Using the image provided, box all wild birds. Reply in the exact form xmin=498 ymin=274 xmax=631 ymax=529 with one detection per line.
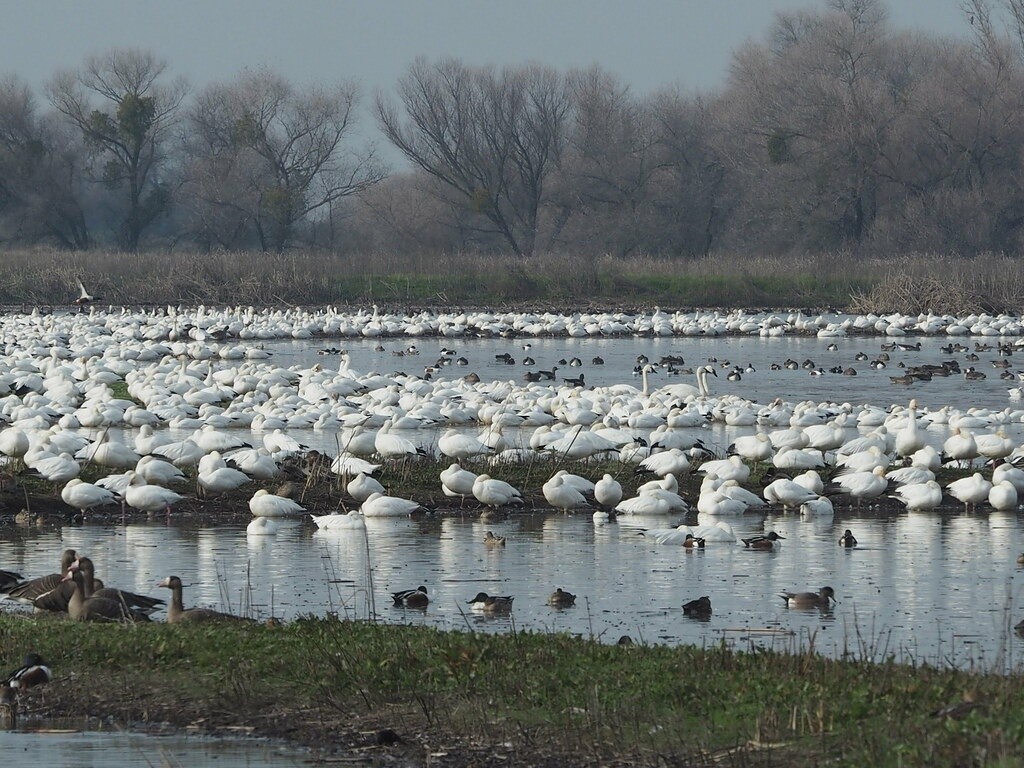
xmin=465 ymin=592 xmax=515 ymax=612
xmin=0 ymin=270 xmax=1024 ymax=551
xmin=548 ymin=588 xmax=577 ymax=604
xmin=390 ymin=586 xmax=429 ymax=610
xmin=777 ymin=587 xmax=836 ymax=609
xmin=0 ymin=549 xmax=256 ymax=629
xmin=618 ymin=636 xmax=634 ymax=649
xmin=0 ymin=653 xmax=52 ymax=700
xmin=681 ymin=597 xmax=712 ymax=621
